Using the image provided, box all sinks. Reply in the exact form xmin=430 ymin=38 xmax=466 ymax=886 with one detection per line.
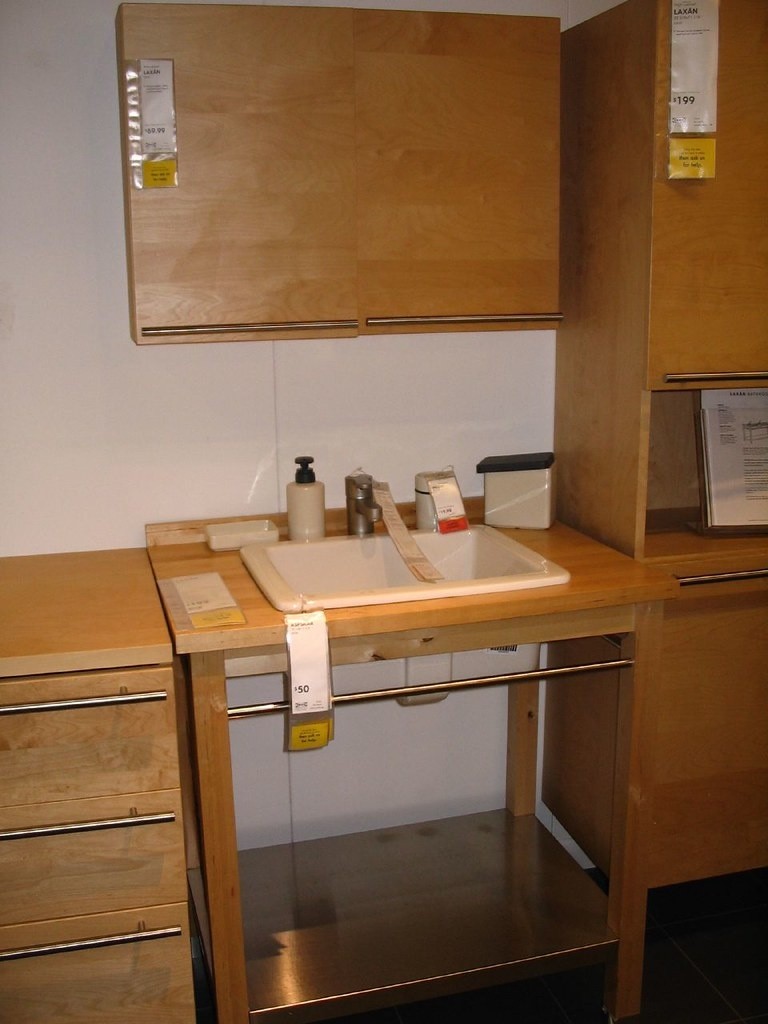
xmin=240 ymin=524 xmax=571 ymax=612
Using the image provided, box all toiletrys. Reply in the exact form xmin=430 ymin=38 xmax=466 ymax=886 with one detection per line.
xmin=286 ymin=456 xmax=325 ymax=540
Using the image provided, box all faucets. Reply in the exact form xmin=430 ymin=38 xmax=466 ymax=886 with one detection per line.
xmin=345 ymin=474 xmax=383 ymax=536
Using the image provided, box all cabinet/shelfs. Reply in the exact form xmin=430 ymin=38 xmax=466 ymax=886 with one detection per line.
xmin=114 ymin=3 xmax=563 ymax=345
xmin=554 ymin=0 xmax=768 ymax=890
xmin=0 ymin=547 xmax=197 ymax=1024
